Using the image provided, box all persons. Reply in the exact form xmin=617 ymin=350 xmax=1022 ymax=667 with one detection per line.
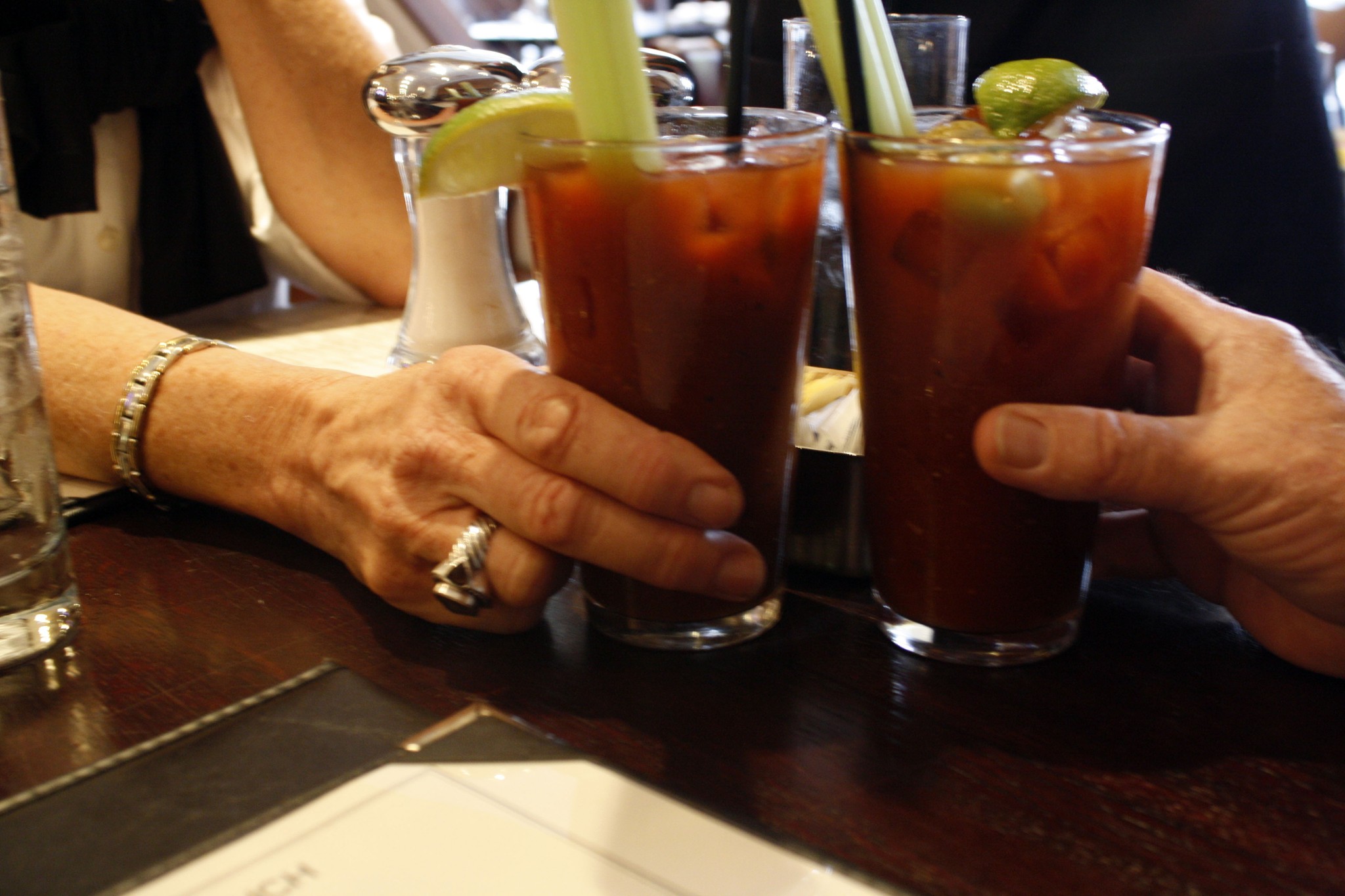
xmin=0 ymin=1 xmax=1345 ymax=683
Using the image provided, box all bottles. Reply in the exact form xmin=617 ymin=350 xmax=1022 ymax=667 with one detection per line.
xmin=365 ymin=47 xmax=545 ymax=377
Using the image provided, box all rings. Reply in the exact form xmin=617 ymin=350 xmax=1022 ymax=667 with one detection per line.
xmin=430 ymin=514 xmax=499 ymax=619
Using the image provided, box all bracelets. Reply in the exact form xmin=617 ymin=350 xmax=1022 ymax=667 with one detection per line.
xmin=108 ymin=336 xmax=235 ymax=502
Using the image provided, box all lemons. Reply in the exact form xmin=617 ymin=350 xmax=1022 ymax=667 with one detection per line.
xmin=417 ymin=86 xmax=591 ymax=198
xmin=974 ymin=57 xmax=1108 ymax=137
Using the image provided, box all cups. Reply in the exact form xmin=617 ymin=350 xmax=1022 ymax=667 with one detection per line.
xmin=780 ymin=12 xmax=969 ymax=370
xmin=518 ymin=104 xmax=832 ymax=649
xmin=833 ymin=109 xmax=1169 ymax=666
xmin=0 ymin=122 xmax=80 ymax=668
